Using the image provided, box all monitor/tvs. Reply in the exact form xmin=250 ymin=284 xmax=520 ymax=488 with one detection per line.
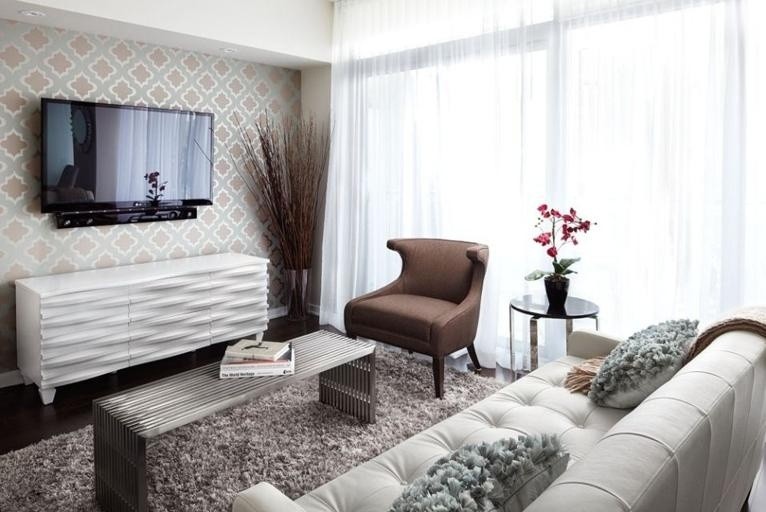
xmin=40 ymin=96 xmax=214 ymax=213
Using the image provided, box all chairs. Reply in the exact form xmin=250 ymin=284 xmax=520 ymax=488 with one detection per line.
xmin=342 ymin=236 xmax=489 ymax=402
xmin=46 ymin=162 xmax=79 ymax=191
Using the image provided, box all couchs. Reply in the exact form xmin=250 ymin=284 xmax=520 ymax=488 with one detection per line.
xmin=226 ymin=308 xmax=763 ymax=512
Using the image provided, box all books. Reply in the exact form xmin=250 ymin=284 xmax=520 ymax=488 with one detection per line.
xmin=218 ymin=339 xmax=295 ymax=380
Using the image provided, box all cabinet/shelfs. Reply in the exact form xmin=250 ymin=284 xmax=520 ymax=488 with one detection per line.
xmin=11 ymin=251 xmax=271 ymax=408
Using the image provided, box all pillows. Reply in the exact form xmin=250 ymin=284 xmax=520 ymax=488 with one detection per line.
xmin=390 ymin=433 xmax=570 ymax=512
xmin=56 ymin=185 xmax=88 ymax=201
xmin=587 ymin=315 xmax=699 ymax=412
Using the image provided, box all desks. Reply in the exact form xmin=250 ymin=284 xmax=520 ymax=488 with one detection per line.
xmin=87 ymin=326 xmax=376 ymax=512
xmin=506 ymin=296 xmax=600 ymax=382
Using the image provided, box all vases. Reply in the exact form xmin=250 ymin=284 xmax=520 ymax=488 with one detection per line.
xmin=542 ymin=275 xmax=569 ymax=309
xmin=284 ymin=266 xmax=313 ymax=322
xmin=149 ymin=200 xmax=161 ymax=206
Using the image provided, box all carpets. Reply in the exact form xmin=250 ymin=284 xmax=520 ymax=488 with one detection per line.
xmin=0 ymin=343 xmax=504 ymax=512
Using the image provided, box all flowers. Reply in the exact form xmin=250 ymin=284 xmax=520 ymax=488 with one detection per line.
xmin=519 ymin=201 xmax=593 ymax=281
xmin=142 ymin=168 xmax=167 ymax=202
